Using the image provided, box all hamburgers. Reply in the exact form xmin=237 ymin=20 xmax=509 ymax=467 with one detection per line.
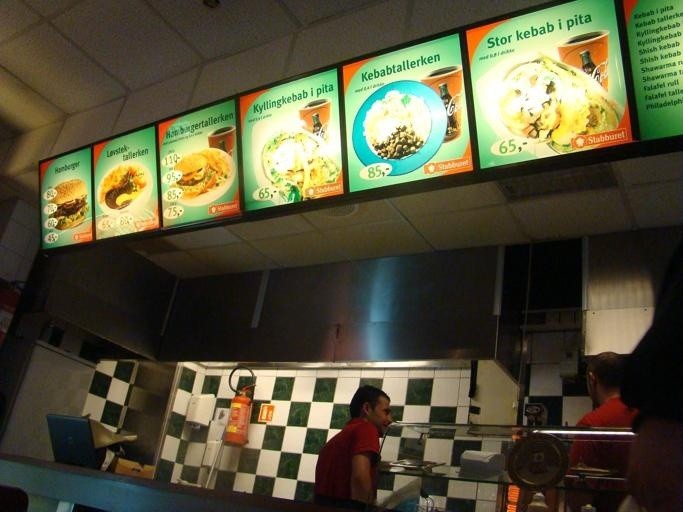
xmin=200 ymin=148 xmax=232 ymax=184
xmin=175 ymin=153 xmax=208 ymax=197
xmin=48 ymin=179 xmax=88 ymax=230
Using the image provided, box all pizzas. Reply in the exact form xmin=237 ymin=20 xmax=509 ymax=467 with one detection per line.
xmin=263 ymin=132 xmax=341 ymax=198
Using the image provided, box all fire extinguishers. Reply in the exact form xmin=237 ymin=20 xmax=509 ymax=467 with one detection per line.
xmin=222 ymin=366 xmax=256 ymax=447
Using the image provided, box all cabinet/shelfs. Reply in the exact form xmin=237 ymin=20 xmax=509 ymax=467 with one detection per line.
xmin=374 ymin=421 xmax=635 ymax=512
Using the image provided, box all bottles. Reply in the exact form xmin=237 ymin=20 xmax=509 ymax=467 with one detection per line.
xmin=437 ymin=84 xmax=461 ymax=141
xmin=579 ymin=50 xmax=601 ymax=82
xmin=311 ymin=113 xmax=323 ymax=138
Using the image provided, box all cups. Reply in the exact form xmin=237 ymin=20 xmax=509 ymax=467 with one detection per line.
xmin=298 ymin=98 xmax=332 ymax=142
xmin=423 ymin=64 xmax=461 ymax=142
xmin=207 ymin=126 xmax=236 ymax=158
xmin=558 ymin=28 xmax=610 ymax=93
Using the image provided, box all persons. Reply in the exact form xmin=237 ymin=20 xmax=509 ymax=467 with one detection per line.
xmin=311 ymin=383 xmax=391 ymax=505
xmin=566 ymin=350 xmax=642 ymax=510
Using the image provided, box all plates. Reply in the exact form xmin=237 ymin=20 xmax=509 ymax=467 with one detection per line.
xmin=170 ymin=147 xmax=235 ymax=208
xmin=97 ymin=161 xmax=154 ymax=214
xmin=487 ymin=54 xmax=625 ymax=154
xmin=352 ymin=80 xmax=448 ymax=175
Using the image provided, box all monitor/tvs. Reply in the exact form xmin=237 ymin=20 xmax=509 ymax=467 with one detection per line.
xmin=46 ymin=414 xmax=107 ymax=470
